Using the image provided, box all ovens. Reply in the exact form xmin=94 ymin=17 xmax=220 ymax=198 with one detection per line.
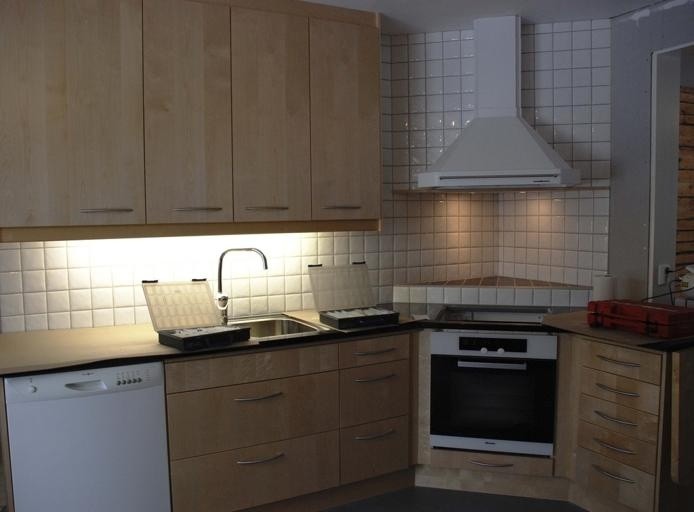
xmin=430 ymin=332 xmax=558 ymax=458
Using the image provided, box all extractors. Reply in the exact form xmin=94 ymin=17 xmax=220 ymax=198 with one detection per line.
xmin=417 ymin=16 xmax=580 ymax=191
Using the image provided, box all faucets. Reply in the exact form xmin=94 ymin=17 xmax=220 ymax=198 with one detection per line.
xmin=217 ymin=248 xmax=267 ymax=295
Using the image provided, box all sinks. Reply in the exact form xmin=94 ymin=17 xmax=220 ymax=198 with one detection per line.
xmin=226 ymin=312 xmax=341 ymax=341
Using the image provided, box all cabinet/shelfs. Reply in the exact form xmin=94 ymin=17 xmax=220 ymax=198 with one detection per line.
xmin=0 ymin=0 xmax=146 ymax=244
xmin=541 ymin=308 xmax=690 ymax=512
xmin=142 ymin=0 xmax=234 ymax=238
xmin=341 ymin=330 xmax=417 ymax=506
xmin=163 ymin=340 xmax=340 ymax=512
xmin=308 ymin=3 xmax=384 ymax=232
xmin=416 ymin=328 xmax=570 ymax=502
xmin=229 ymin=0 xmax=312 ymax=235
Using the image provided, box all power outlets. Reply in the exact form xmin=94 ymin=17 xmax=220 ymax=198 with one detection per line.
xmin=657 ymin=264 xmax=671 ymax=285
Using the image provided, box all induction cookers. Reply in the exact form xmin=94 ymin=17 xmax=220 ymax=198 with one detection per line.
xmin=434 ymin=303 xmax=552 ymax=330
